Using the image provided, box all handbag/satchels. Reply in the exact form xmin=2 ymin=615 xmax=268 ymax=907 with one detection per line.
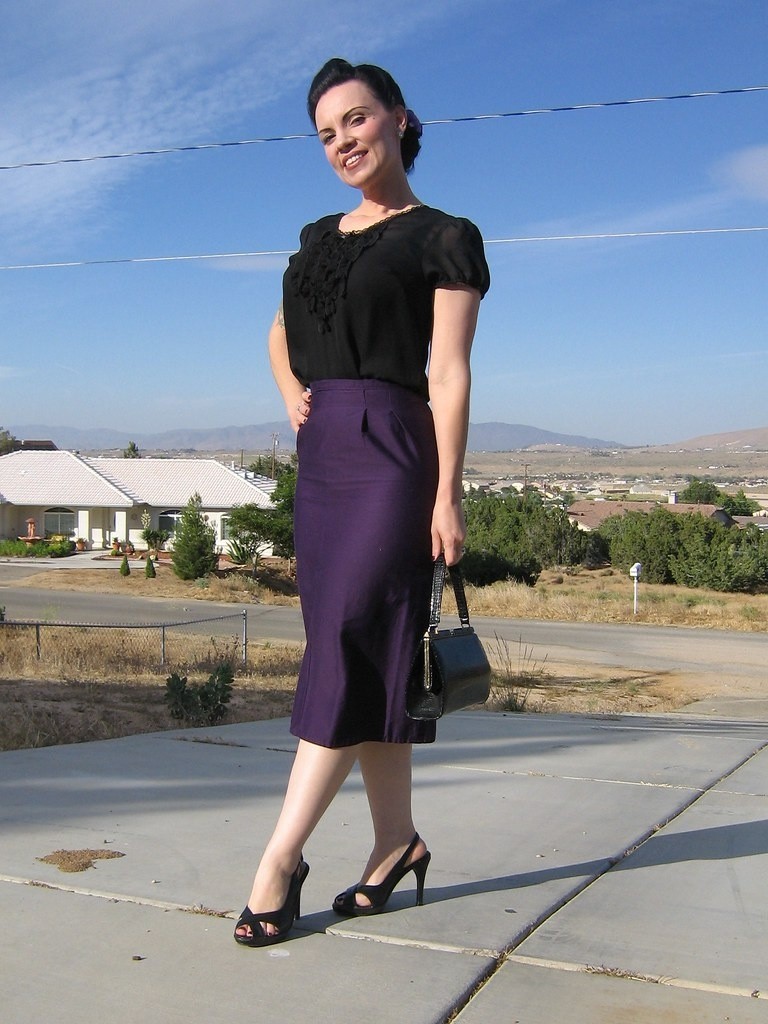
xmin=404 ymin=554 xmax=492 ymax=721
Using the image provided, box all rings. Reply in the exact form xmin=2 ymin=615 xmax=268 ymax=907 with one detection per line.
xmin=298 ymin=403 xmax=306 ymax=411
xmin=461 ymin=547 xmax=466 ymax=554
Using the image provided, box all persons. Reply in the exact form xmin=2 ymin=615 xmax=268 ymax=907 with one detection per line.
xmin=234 ymin=58 xmax=490 ymax=948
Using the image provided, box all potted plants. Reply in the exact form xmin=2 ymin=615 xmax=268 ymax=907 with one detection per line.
xmin=75 ymin=538 xmax=86 ymax=551
xmin=112 ymin=538 xmax=121 ymax=551
xmin=125 ymin=541 xmax=135 ymax=553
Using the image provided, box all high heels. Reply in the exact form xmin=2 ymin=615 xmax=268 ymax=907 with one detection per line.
xmin=332 ymin=832 xmax=431 ymax=917
xmin=234 ymin=853 xmax=310 ymax=948
